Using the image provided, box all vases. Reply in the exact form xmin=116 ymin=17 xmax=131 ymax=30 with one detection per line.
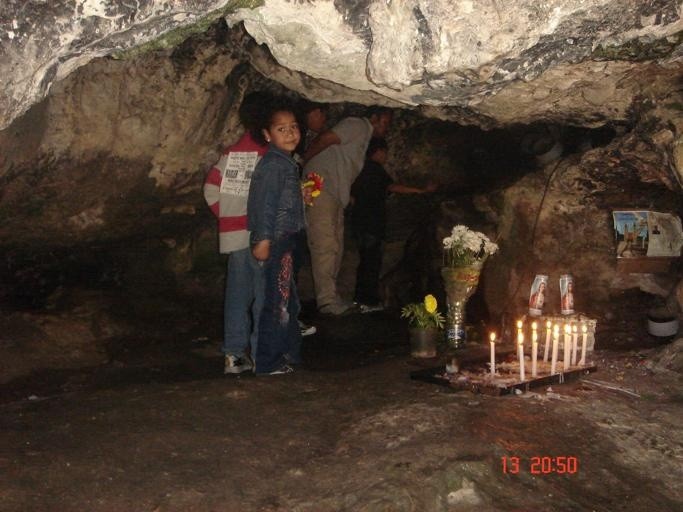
xmin=445 ymin=298 xmax=466 ymax=351
xmin=408 ymin=324 xmax=437 ymax=358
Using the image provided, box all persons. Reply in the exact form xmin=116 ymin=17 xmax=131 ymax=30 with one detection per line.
xmin=529 ymin=282 xmax=546 ymax=309
xmin=203 ymin=91 xmax=275 ymax=374
xmin=563 ymin=281 xmax=574 ymax=309
xmin=246 ymin=102 xmax=303 ymax=378
xmin=298 ymin=109 xmax=393 ymax=318
xmin=650 ymin=225 xmax=661 ymax=234
xmin=297 ymin=104 xmax=355 ymax=207
xmin=349 ymin=137 xmax=439 ymax=315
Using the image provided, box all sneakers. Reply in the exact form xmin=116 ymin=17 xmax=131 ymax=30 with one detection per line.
xmin=296 ymin=319 xmax=318 ymax=337
xmin=223 ymin=350 xmax=295 ymax=375
xmin=318 ymin=298 xmax=386 ymax=318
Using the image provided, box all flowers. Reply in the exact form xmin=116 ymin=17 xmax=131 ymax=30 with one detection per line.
xmin=397 ymin=293 xmax=446 ymax=346
xmin=440 ymin=224 xmax=498 ymax=325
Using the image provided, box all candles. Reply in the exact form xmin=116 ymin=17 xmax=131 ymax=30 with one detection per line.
xmin=487 ymin=315 xmax=588 ymax=380
